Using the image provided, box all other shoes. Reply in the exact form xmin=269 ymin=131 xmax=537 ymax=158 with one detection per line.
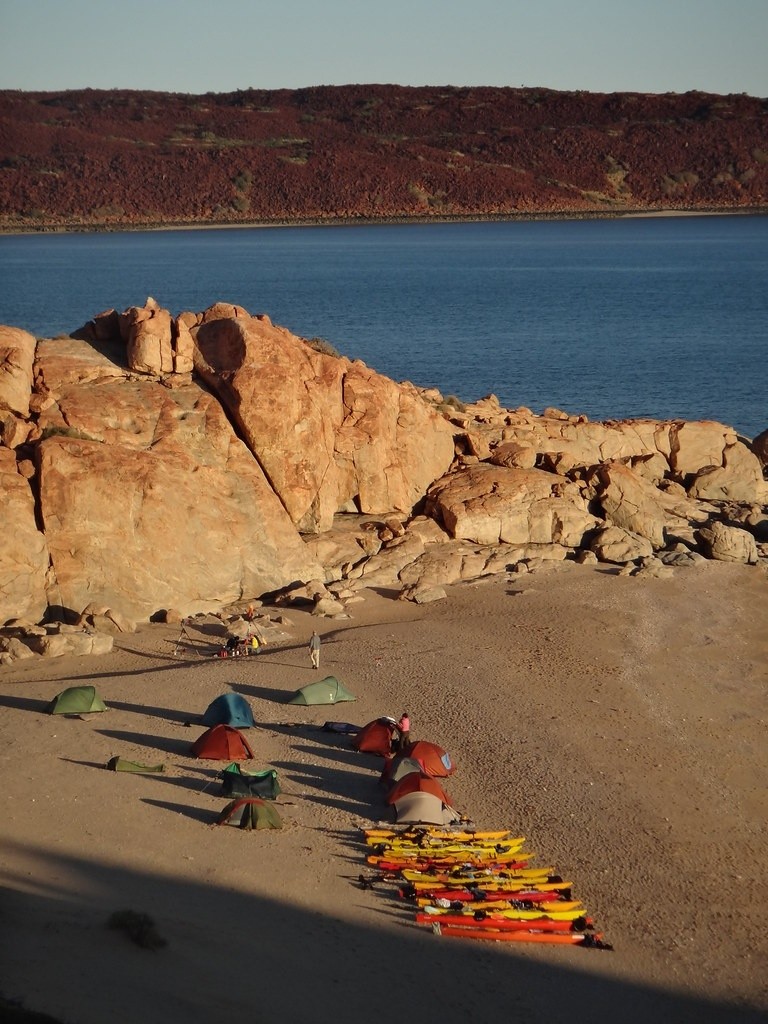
xmin=312 ymin=665 xmax=318 ymax=669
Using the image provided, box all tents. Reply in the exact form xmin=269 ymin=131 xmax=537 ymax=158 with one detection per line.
xmin=373 ymin=740 xmax=473 ymax=826
xmin=217 ymin=797 xmax=283 ymax=830
xmin=200 ymin=762 xmax=283 ymax=800
xmin=49 ymin=686 xmax=109 ymax=714
xmin=192 ymin=724 xmax=256 ymax=761
xmin=287 ymin=676 xmax=357 ymax=706
xmin=201 ymin=692 xmax=256 ymax=728
xmin=106 ymin=756 xmax=166 ymax=772
xmin=351 ymin=718 xmax=412 ymax=757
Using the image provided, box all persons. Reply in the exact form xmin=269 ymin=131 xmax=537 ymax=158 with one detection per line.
xmin=399 ymin=713 xmax=410 ymax=751
xmin=227 ymin=636 xmax=241 ymax=652
xmin=309 ymin=631 xmax=320 ymax=669
xmin=244 ymin=634 xmax=262 ymax=656
